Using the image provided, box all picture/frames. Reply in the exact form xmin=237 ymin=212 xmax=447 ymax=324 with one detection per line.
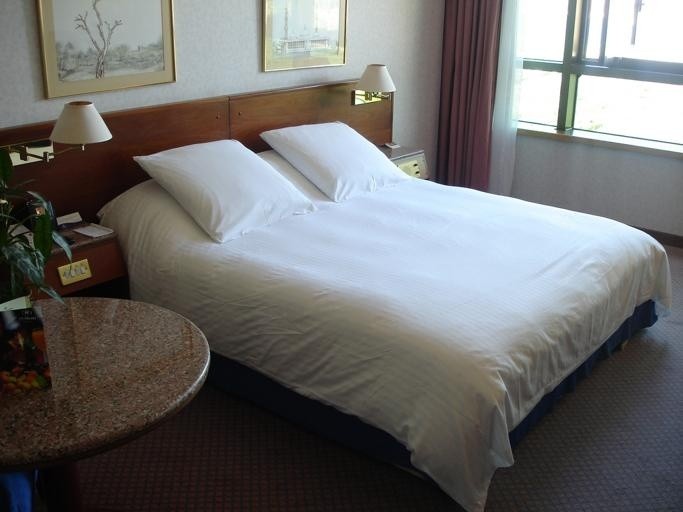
xmin=260 ymin=1 xmax=348 ymax=73
xmin=35 ymin=0 xmax=176 ymax=101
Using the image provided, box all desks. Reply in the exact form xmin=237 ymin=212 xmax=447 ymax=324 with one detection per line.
xmin=0 ymin=296 xmax=211 ymax=512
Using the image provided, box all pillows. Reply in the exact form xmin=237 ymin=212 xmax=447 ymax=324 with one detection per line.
xmin=132 ymin=121 xmax=410 ymax=244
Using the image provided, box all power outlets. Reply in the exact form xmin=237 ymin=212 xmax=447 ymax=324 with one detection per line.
xmin=0 ymin=101 xmax=112 ymax=168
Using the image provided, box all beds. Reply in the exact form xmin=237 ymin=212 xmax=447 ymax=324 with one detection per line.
xmin=98 ymin=147 xmax=660 ymax=496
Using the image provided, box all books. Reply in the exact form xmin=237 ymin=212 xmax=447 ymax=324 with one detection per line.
xmin=73 ymin=221 xmax=114 ymax=239
xmin=8 ymin=223 xmax=34 ymax=251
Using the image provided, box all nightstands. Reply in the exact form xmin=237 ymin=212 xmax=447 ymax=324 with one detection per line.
xmin=21 ymin=220 xmax=127 ymax=298
xmin=376 ymin=144 xmax=430 ymax=181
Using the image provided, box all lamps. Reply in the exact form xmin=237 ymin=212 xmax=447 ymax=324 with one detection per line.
xmin=350 ymin=64 xmax=397 ymax=107
